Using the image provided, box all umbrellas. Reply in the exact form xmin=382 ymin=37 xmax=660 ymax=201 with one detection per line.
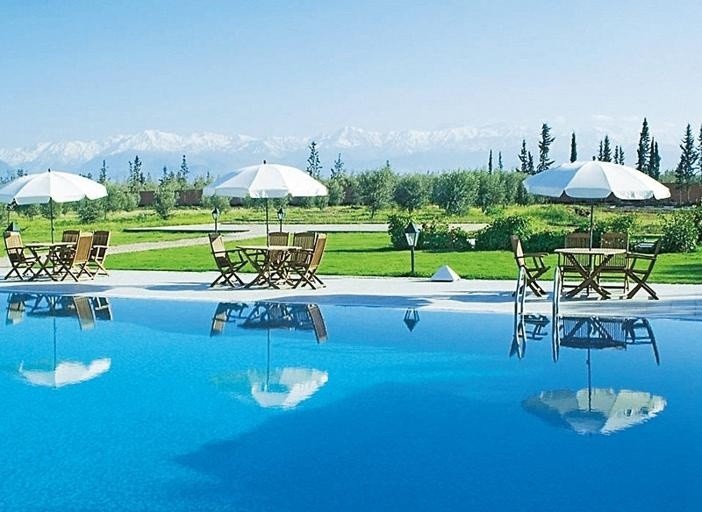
xmin=3 ymin=316 xmax=112 ymax=391
xmin=213 ymin=300 xmax=329 ymax=411
xmin=520 ymin=320 xmax=667 ymax=438
xmin=201 ymin=159 xmax=328 ymax=246
xmin=523 ymin=156 xmax=671 ymax=276
xmin=0 ymin=168 xmax=108 ymax=245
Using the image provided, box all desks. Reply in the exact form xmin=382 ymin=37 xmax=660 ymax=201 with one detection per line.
xmin=24 ymin=242 xmax=76 ymax=280
xmin=28 ymin=297 xmax=76 ymax=318
xmin=236 ymin=246 xmax=302 ymax=290
xmin=559 ymin=320 xmax=628 ymax=350
xmin=236 ymin=303 xmax=295 ymax=330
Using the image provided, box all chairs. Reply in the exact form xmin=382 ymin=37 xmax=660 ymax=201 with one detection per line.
xmin=287 ymin=233 xmax=327 ymax=290
xmin=208 ymin=232 xmax=248 ymax=289
xmin=281 ymin=232 xmax=317 ymax=285
xmin=257 ymin=232 xmax=288 ymax=283
xmin=509 ymin=315 xmax=550 ymax=359
xmin=290 ymin=304 xmax=329 ymax=344
xmin=59 ymin=235 xmax=93 ymax=282
xmin=52 ymin=229 xmax=80 ymax=274
xmin=621 ymin=318 xmax=660 ymax=367
xmin=507 ymin=232 xmax=663 ymax=301
xmin=56 ymin=296 xmax=95 ymax=331
xmin=5 ymin=292 xmax=39 ymax=325
xmin=89 ymin=296 xmax=113 ymax=322
xmin=210 ymin=302 xmax=249 ymax=338
xmin=3 ymin=231 xmax=41 ymax=280
xmin=82 ymin=231 xmax=111 ymax=279
